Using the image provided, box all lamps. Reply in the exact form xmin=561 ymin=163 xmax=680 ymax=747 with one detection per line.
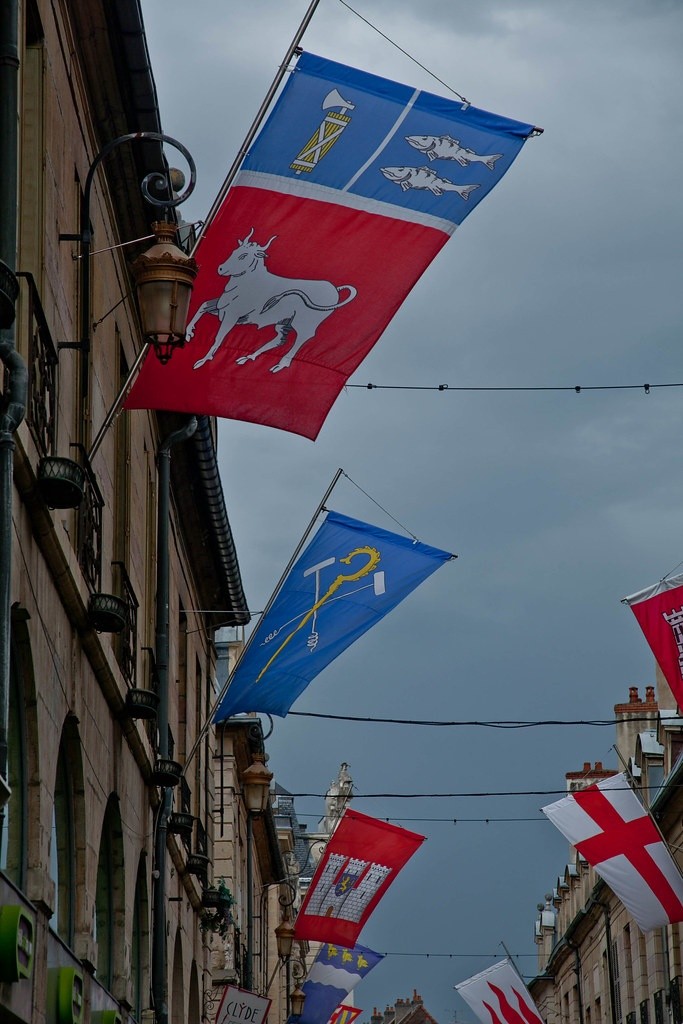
xmin=260 ymin=878 xmax=298 ymax=964
xmin=237 ymin=729 xmax=273 ymax=820
xmin=57 ymin=126 xmax=201 ymax=389
xmin=279 ymin=958 xmax=306 ymax=1016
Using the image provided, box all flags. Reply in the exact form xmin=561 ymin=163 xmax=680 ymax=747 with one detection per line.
xmin=293 ymin=808 xmax=426 ymax=949
xmin=626 ymin=572 xmax=683 ymax=713
xmin=287 ymin=942 xmax=385 ymax=1024
xmin=542 ymin=774 xmax=683 ymax=935
xmin=455 ymin=958 xmax=544 ymax=1024
xmin=125 ymin=51 xmax=538 ymax=444
xmin=326 ymin=1004 xmax=363 ymax=1024
xmin=213 ymin=512 xmax=452 ymax=724
